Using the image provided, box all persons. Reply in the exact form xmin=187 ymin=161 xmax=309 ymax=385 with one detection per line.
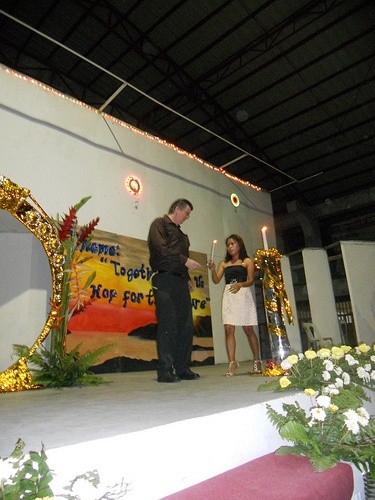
xmin=208 ymin=234 xmax=262 ymax=376
xmin=148 ymin=199 xmax=200 ymax=383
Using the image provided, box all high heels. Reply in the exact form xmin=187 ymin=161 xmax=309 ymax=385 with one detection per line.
xmin=252 ymin=360 xmax=262 ymax=375
xmin=226 ymin=361 xmax=239 ymax=377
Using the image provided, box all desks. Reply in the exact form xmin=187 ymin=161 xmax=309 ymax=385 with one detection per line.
xmin=158 ymin=446 xmax=375 ymax=500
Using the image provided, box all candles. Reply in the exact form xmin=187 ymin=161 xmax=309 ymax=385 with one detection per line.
xmin=261 ymin=226 xmax=269 ymax=251
xmin=211 ymin=240 xmax=218 ymax=264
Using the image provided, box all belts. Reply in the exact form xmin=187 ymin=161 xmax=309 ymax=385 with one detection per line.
xmin=154 ymin=270 xmax=185 ymax=278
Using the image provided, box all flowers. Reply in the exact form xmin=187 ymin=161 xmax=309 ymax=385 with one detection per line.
xmin=266 ymin=342 xmax=375 ymax=473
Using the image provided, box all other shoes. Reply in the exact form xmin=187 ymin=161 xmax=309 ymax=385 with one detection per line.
xmin=156 ymin=365 xmax=181 ymax=382
xmin=175 ymin=364 xmax=200 ymax=379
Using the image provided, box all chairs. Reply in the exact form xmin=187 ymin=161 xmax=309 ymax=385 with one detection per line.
xmin=302 ymin=322 xmax=334 ymax=350
